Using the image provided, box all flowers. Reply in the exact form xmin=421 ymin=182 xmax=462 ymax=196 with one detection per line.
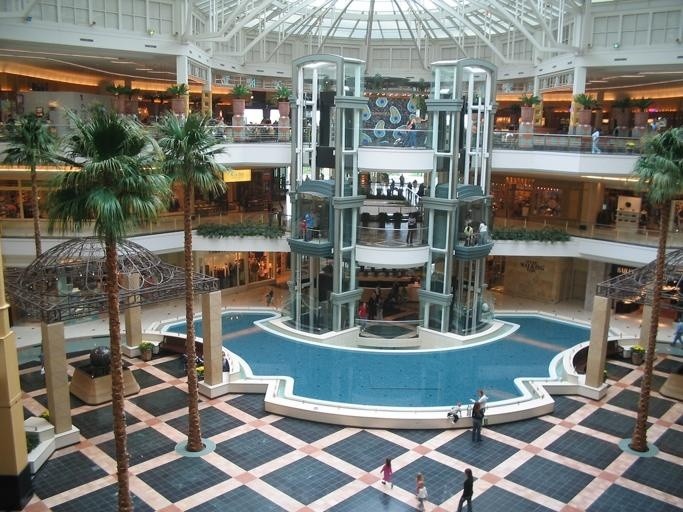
xmin=630 ymin=345 xmax=644 ymax=354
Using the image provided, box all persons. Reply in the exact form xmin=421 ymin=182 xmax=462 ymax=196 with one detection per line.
xmin=671 ymin=319 xmax=682 ymax=346
xmin=475 ymin=391 xmax=488 ymax=415
xmin=478 ymin=220 xmax=486 ymax=246
xmin=464 ymin=223 xmax=473 ymax=248
xmin=249 ymin=120 xmax=276 ymax=139
xmin=407 ymin=214 xmax=416 ymax=243
xmin=447 ymin=403 xmax=462 ymax=424
xmin=305 ymin=174 xmax=310 ymax=181
xmin=415 ymin=473 xmax=427 ymax=511
xmin=456 ymin=468 xmax=473 ymax=511
xmin=482 ymin=302 xmax=488 ymax=313
xmin=590 ymin=127 xmax=600 ymax=153
xmin=405 ymin=118 xmax=414 ymax=147
xmin=360 ymin=302 xmax=367 ymax=333
xmin=380 ymin=457 xmax=393 ymax=488
xmin=388 ymin=173 xmax=417 ymax=196
xmin=368 ymin=292 xmax=376 ymax=319
xmin=376 ymin=289 xmax=384 ymax=320
xmin=471 ymin=402 xmax=481 ymax=442
xmin=320 ymin=173 xmax=324 ymax=180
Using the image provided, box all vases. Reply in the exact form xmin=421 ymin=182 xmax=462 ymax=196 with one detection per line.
xmin=631 ymin=351 xmax=643 ymax=365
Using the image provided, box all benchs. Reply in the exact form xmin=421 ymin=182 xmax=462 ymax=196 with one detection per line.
xmin=158 ymin=334 xmax=203 ymax=361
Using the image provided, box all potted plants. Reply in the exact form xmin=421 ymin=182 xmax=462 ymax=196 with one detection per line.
xmin=518 ymin=91 xmax=544 ymax=122
xmin=164 ymin=81 xmax=192 ymax=113
xmin=633 ymin=92 xmax=655 ymax=124
xmin=137 ymin=341 xmax=155 ymax=361
xmin=271 ymin=81 xmax=293 ymax=115
xmin=572 ymin=92 xmax=598 ymax=124
xmin=226 ymin=83 xmax=253 ymax=114
